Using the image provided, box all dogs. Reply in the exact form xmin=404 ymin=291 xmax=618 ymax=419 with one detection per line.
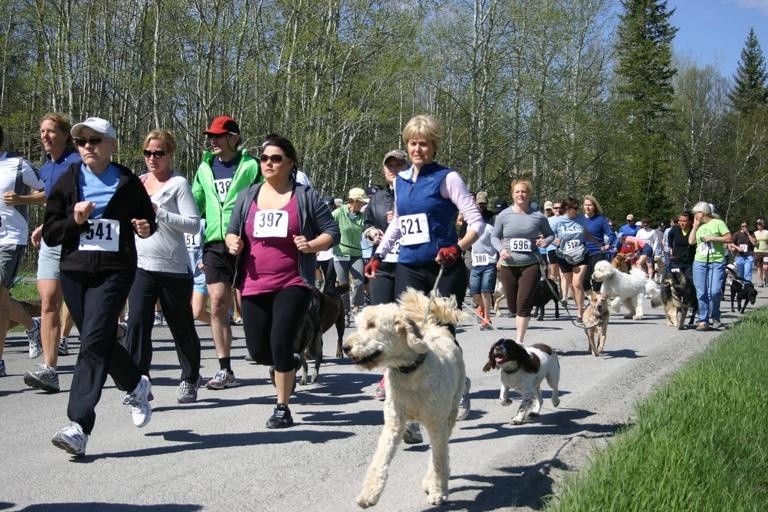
xmin=270 ymin=282 xmax=351 ymax=396
xmin=755 ymin=257 xmax=768 ymax=288
xmin=340 ymin=286 xmax=466 ymax=509
xmin=532 ymin=279 xmax=559 ymax=321
xmin=591 ymin=252 xmax=699 ymax=330
xmin=483 ymin=339 xmax=560 ymax=425
xmin=582 ymin=289 xmax=610 ymax=356
xmin=725 ymin=267 xmax=757 ymax=314
xmin=493 ymin=278 xmax=506 ymax=317
xmin=725 ymin=262 xmax=737 ymax=286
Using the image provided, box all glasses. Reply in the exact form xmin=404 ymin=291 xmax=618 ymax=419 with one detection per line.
xmin=143 ymin=149 xmax=165 ymax=159
xmin=207 ymin=133 xmax=222 ymax=139
xmin=260 ymin=154 xmax=283 ymax=162
xmin=72 ymin=136 xmax=102 ymax=147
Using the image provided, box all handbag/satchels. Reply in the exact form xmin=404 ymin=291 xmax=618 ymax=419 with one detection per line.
xmin=563 ymin=244 xmax=585 ymax=266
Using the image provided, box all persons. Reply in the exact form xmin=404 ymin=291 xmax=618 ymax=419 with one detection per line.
xmin=456 ymin=181 xmax=661 ymax=346
xmin=314 ymin=150 xmax=412 ymax=401
xmin=363 ymin=114 xmax=485 ymax=444
xmin=0 ymin=113 xmax=151 ymax=457
xmin=122 ymin=115 xmax=341 ymax=428
xmin=655 ymin=202 xmax=768 ymax=330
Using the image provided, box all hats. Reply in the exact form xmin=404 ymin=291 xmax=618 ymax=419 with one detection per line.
xmin=348 ymin=187 xmax=370 ymax=203
xmin=201 ymin=115 xmax=240 ymax=135
xmin=475 ymin=191 xmax=487 ymax=204
xmin=492 ymin=199 xmax=508 ymax=213
xmin=543 ymin=200 xmax=553 ymax=211
xmin=365 ymin=186 xmax=378 ymax=197
xmin=382 ymin=149 xmax=408 ymax=166
xmin=69 ymin=116 xmax=116 ymax=140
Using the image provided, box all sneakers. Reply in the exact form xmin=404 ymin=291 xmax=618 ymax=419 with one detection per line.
xmin=402 ymin=423 xmax=424 ymax=445
xmin=479 ymin=318 xmax=492 ymax=331
xmin=695 ymin=321 xmax=708 ymax=331
xmin=376 ymin=378 xmax=385 ymax=403
xmin=476 ymin=305 xmax=484 ymax=323
xmin=0 ymin=317 xmax=68 ymax=392
xmin=121 ymin=374 xmax=152 ymax=427
xmin=265 ymin=402 xmax=293 ymax=429
xmin=713 ymin=320 xmax=723 ymax=329
xmin=154 ymin=310 xmax=164 ymax=326
xmin=205 ymin=368 xmax=236 ymax=389
xmin=118 ymin=322 xmax=127 ymax=348
xmin=175 ymin=374 xmax=203 ymax=403
xmin=52 ymin=422 xmax=89 ymax=459
xmin=456 ymin=377 xmax=471 ymax=422
